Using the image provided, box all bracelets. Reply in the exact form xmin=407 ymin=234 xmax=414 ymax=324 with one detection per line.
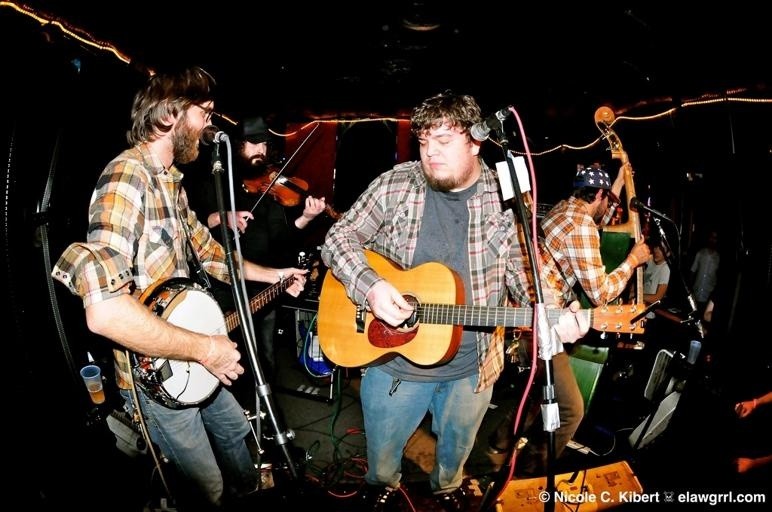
xmin=752 ymin=398 xmax=758 ymax=409
xmin=628 ymin=252 xmax=639 ymax=267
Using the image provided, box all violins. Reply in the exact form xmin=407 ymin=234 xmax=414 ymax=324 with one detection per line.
xmin=243 ymin=164 xmax=345 ymax=221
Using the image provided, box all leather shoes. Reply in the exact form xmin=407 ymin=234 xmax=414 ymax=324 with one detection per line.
xmin=366 ymin=487 xmax=398 ymax=510
xmin=436 ymin=485 xmax=471 ymax=510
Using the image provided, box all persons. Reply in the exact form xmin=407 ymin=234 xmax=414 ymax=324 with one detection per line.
xmin=81 ymin=63 xmax=310 ymax=504
xmin=734 ymin=454 xmax=772 ymax=475
xmin=643 ymin=239 xmax=672 ymax=304
xmin=688 ymin=230 xmax=723 ymax=304
xmin=733 ymin=391 xmax=772 ymax=418
xmin=479 ymin=161 xmax=651 ymax=477
xmin=319 ymin=86 xmax=595 ymax=510
xmin=187 ymin=114 xmax=327 ymax=369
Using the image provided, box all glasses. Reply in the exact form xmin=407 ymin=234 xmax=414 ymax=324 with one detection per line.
xmin=176 ymin=94 xmax=215 ymax=124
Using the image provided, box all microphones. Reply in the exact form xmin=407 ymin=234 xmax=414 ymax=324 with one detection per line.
xmin=470 ymin=108 xmax=511 ymax=142
xmin=200 ymin=125 xmax=226 ymax=147
xmin=630 ymin=197 xmax=671 ymax=223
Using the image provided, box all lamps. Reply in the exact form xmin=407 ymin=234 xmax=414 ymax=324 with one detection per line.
xmin=398 ymin=0 xmax=443 ymax=32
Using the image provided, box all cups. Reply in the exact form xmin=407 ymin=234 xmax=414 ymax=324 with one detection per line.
xmin=687 ymin=340 xmax=701 ymax=365
xmin=80 ymin=365 xmax=106 ymax=404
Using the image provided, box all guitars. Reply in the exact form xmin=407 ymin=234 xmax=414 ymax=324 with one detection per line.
xmin=317 ymin=251 xmax=649 ymax=368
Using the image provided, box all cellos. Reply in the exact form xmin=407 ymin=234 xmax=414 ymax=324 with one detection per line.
xmin=568 ymin=107 xmax=646 ymax=414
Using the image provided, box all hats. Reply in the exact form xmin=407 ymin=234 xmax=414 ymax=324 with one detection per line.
xmin=574 ymin=167 xmax=622 ymax=204
xmin=234 ymin=114 xmax=272 ymax=143
